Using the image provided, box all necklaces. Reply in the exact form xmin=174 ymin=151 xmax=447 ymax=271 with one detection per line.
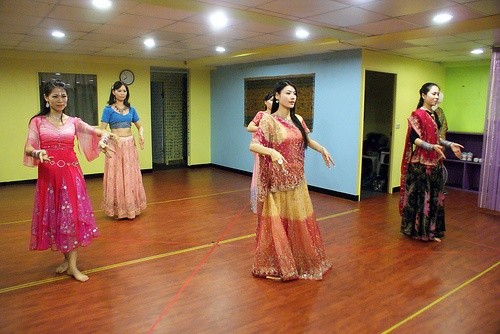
xmin=425 ymin=108 xmax=434 ymax=113
xmin=114 ymin=106 xmax=129 ymax=112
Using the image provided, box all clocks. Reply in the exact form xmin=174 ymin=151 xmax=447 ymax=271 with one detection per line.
xmin=119 ymin=69 xmax=135 ymax=86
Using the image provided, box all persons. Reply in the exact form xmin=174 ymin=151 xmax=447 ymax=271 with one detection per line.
xmin=98 ymin=80 xmax=147 ymax=219
xmin=249 ymin=79 xmax=335 ymax=282
xmin=399 ymin=84 xmax=464 ymax=242
xmin=247 ymin=92 xmax=274 ymax=213
xmin=24 ymin=77 xmax=116 ymax=282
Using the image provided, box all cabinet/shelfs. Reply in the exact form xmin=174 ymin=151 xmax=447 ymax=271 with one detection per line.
xmin=443 ymin=130 xmax=483 ymax=193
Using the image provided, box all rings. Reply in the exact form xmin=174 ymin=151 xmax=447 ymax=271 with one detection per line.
xmin=279 ymin=159 xmax=284 ymax=164
